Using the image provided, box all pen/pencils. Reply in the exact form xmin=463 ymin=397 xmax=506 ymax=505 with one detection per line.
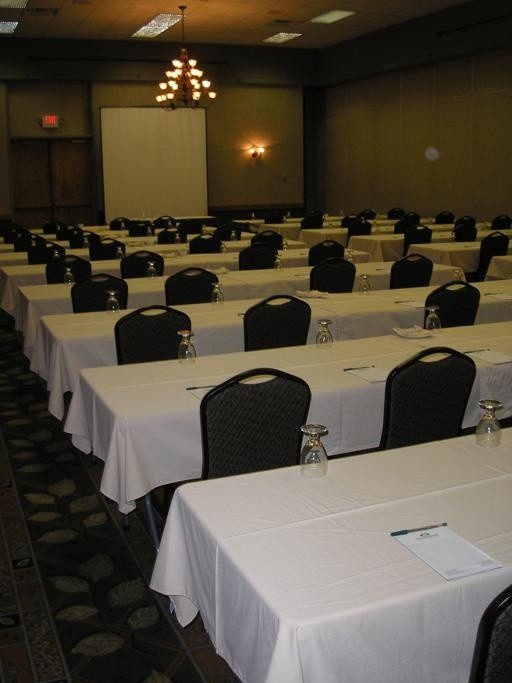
xmin=395 ymin=300 xmax=415 ymax=303
xmin=186 ymin=386 xmax=217 ymax=390
xmin=464 ymin=349 xmax=490 ymax=353
xmin=344 ymin=365 xmax=374 ymax=371
xmin=484 ymin=292 xmax=503 ymax=295
xmin=391 ymin=523 xmax=447 ymax=536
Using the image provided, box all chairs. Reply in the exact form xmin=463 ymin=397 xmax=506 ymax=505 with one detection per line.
xmin=308 ymin=240 xmax=345 ymax=267
xmin=346 ymin=218 xmax=372 ymax=249
xmin=69 ymin=231 xmax=101 ymax=249
xmin=378 ymin=347 xmax=476 ymax=452
xmin=264 ymin=213 xmax=284 ymax=224
xmin=120 ymin=250 xmax=165 ymax=278
xmin=89 ymin=237 xmax=126 ymax=261
xmin=127 ymin=220 xmax=155 ymax=237
xmin=115 ymin=305 xmax=191 ymax=365
xmin=387 ymin=207 xmax=405 ymax=219
xmin=14 ymin=234 xmax=47 ymax=253
xmin=27 ymin=241 xmax=66 ymax=265
xmin=151 ymin=216 xmax=176 ymax=236
xmin=389 ymin=254 xmax=433 ymax=290
xmin=42 ymin=221 xmax=64 ymax=235
xmin=393 ymin=217 xmax=418 ymax=234
xmin=401 ymin=212 xmax=421 ymax=226
xmin=301 ymin=215 xmax=323 ymax=230
xmin=165 ymin=266 xmax=219 ymax=305
xmin=200 ymin=367 xmax=312 ymax=482
xmin=3 ymin=226 xmax=31 ymax=245
xmin=110 ymin=217 xmax=130 ymax=230
xmin=357 ymin=208 xmax=376 ymax=220
xmin=213 ymin=224 xmax=242 ymax=241
xmin=189 ymin=233 xmax=223 ymax=254
xmin=56 ymin=224 xmax=82 ymax=240
xmin=70 ymin=272 xmax=128 ymax=314
xmin=469 ymin=582 xmax=512 ymax=682
xmin=454 ymin=215 xmax=477 ymax=232
xmin=341 ymin=216 xmax=365 ymax=229
xmin=45 ymin=254 xmax=93 ymax=286
xmin=403 ymin=224 xmax=432 ymax=258
xmin=242 ymin=295 xmax=312 ymax=352
xmin=424 ymin=279 xmax=481 ymax=331
xmin=157 ymin=225 xmax=188 ymax=245
xmin=310 ymin=259 xmax=357 ymax=296
xmin=251 ymin=231 xmax=283 ymax=249
xmin=454 ymin=223 xmax=477 ymax=244
xmin=477 ymin=231 xmax=510 ymax=275
xmin=238 ymin=242 xmax=278 ymax=271
xmin=435 ymin=211 xmax=455 ymax=225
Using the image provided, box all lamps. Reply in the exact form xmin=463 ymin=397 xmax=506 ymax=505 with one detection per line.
xmin=156 ymin=5 xmax=217 ymax=112
xmin=249 ymin=144 xmax=263 ymax=160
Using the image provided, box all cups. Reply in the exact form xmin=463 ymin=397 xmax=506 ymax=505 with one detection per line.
xmin=476 ymin=398 xmax=504 ymax=449
xmin=359 ymin=274 xmax=371 ymax=295
xmin=425 ymin=306 xmax=441 ymax=336
xmin=301 ymin=424 xmax=329 ymax=478
xmin=106 ymin=290 xmax=119 ymax=314
xmin=147 ymin=261 xmax=157 ymax=277
xmin=64 ymin=267 xmax=73 ymax=286
xmin=177 ymin=330 xmax=197 ymax=365
xmin=316 ymin=319 xmax=333 ymax=350
xmin=211 ymin=283 xmax=224 ymax=304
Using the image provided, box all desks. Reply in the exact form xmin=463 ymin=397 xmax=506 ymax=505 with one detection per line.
xmin=406 ymin=240 xmax=512 ymax=273
xmin=14 ymin=260 xmax=466 ymax=361
xmin=64 ymin=319 xmax=511 ymax=529
xmin=0 ymin=237 xmax=306 ymax=267
xmin=487 ymin=255 xmax=512 ymax=283
xmin=348 ymin=229 xmax=512 ymax=261
xmin=30 ymin=278 xmax=511 ymax=420
xmin=300 ymin=222 xmax=493 ymax=247
xmin=0 ymin=247 xmax=371 ymax=316
xmin=258 ymin=218 xmax=457 ymax=241
xmin=149 ymin=426 xmax=512 ymax=681
